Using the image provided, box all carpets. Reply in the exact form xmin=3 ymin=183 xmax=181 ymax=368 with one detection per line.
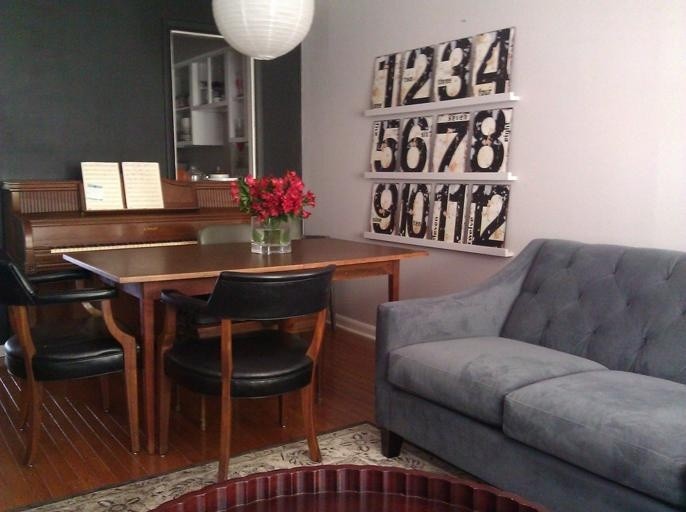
xmin=10 ymin=419 xmax=480 ymax=512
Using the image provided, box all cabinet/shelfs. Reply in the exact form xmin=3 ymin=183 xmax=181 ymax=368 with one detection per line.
xmin=173 ymin=58 xmax=224 ymax=148
xmin=363 ymin=92 xmax=519 ymax=258
xmin=191 ymin=47 xmax=226 ymax=111
xmin=225 ymin=47 xmax=252 ymax=142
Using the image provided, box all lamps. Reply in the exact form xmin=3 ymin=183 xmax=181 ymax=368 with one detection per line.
xmin=209 ymin=0 xmax=314 ymax=61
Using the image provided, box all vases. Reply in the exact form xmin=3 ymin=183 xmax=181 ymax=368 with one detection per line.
xmin=250 ymin=215 xmax=292 ymax=254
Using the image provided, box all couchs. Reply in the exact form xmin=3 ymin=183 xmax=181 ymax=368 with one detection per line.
xmin=374 ymin=239 xmax=685 ymax=511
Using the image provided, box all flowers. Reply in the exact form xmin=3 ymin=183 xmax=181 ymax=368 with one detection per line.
xmin=229 ymin=167 xmax=320 ymax=244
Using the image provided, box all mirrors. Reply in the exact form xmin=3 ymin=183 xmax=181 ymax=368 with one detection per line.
xmin=159 ymin=17 xmax=265 ymax=185
